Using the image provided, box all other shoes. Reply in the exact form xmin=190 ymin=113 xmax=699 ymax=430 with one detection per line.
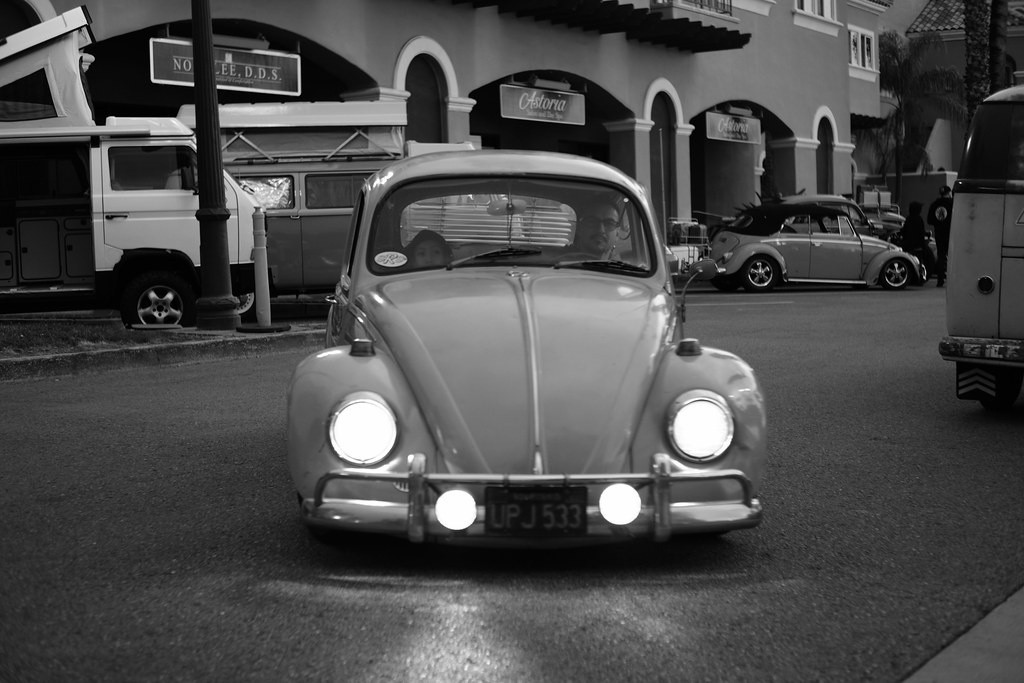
xmin=936 ymin=279 xmax=944 ymax=287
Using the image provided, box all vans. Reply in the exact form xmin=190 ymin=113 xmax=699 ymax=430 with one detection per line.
xmin=938 ymin=85 xmax=1024 ymax=410
xmin=0 ymin=115 xmax=268 ymax=328
xmin=222 ymin=149 xmax=402 ymax=322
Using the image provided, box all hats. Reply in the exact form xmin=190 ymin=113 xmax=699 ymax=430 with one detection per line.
xmin=939 ymin=186 xmax=951 ymax=195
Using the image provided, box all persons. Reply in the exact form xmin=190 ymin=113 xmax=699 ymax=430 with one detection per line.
xmin=900 ymin=185 xmax=953 ymax=288
xmin=402 ymin=229 xmax=453 ymax=270
xmin=580 ymin=195 xmax=649 ymax=268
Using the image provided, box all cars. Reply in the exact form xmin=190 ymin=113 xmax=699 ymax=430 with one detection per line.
xmin=664 ymin=195 xmax=937 ymax=291
xmin=286 ymin=150 xmax=767 ymax=550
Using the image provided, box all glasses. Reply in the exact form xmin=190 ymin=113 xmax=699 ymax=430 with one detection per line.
xmin=579 ymin=216 xmax=620 ymax=232
